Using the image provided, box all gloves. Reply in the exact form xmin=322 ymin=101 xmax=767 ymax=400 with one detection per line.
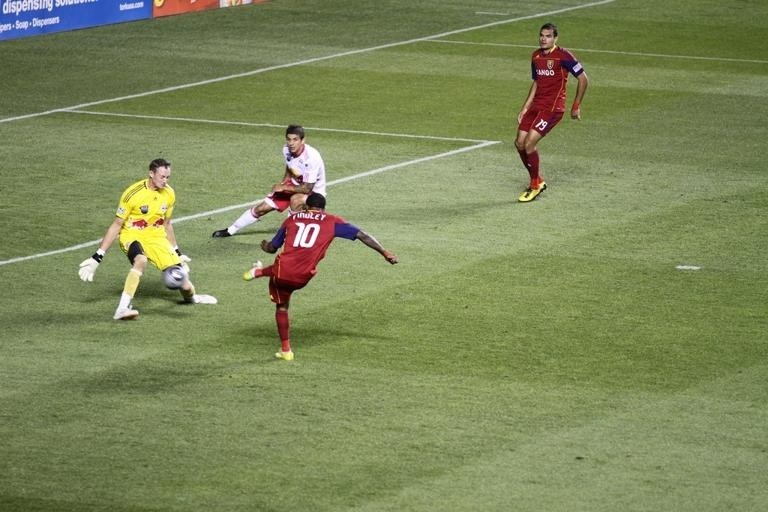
xmin=175 ymin=247 xmax=191 ymax=273
xmin=79 ymin=248 xmax=106 ymax=282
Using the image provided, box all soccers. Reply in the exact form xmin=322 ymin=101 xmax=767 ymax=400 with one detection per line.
xmin=163 ymin=266 xmax=185 ymax=290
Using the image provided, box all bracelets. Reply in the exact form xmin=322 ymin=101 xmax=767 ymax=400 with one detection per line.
xmin=572 ymin=102 xmax=579 ymax=111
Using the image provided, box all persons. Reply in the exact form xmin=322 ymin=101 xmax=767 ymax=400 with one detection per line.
xmin=242 ymin=192 xmax=399 ymax=361
xmin=79 ymin=158 xmax=219 ymax=320
xmin=515 ymin=22 xmax=589 ymax=201
xmin=213 ymin=125 xmax=326 ymax=238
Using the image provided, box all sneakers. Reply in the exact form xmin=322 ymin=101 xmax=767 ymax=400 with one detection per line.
xmin=243 ymin=261 xmax=263 ymax=281
xmin=213 ymin=228 xmax=233 ymax=238
xmin=519 ymin=183 xmax=544 ymax=202
xmin=113 ymin=308 xmax=139 ymax=320
xmin=192 ymin=294 xmax=218 ymax=305
xmin=275 ymin=349 xmax=295 ymax=361
